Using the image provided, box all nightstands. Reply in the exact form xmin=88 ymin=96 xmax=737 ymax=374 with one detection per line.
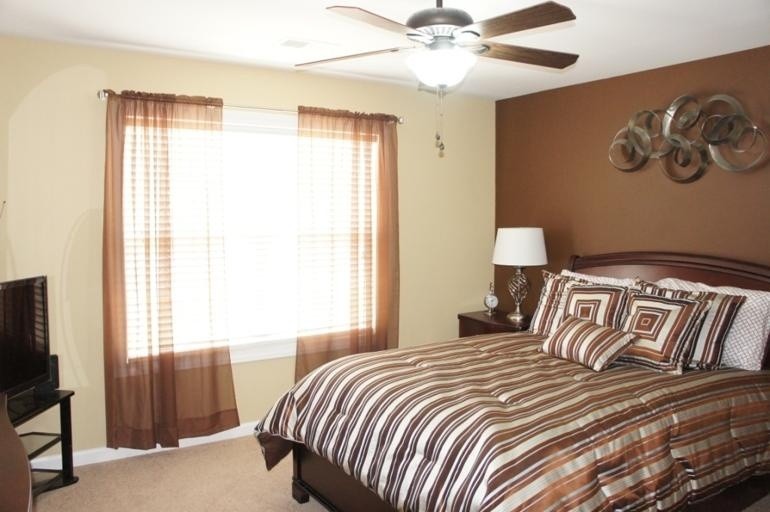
xmin=458 ymin=311 xmax=532 ymax=337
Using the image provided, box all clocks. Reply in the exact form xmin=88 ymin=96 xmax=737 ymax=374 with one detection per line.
xmin=485 ymin=279 xmax=500 ymax=317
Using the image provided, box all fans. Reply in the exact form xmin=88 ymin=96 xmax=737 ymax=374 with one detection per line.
xmin=294 ymin=0 xmax=579 ymax=69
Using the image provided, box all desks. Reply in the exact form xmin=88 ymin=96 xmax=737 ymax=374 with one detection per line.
xmin=6 ymin=389 xmax=78 ymax=497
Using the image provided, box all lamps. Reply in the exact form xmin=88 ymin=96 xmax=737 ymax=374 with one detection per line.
xmin=492 ymin=227 xmax=548 ymax=322
xmin=401 ymin=36 xmax=478 ymax=92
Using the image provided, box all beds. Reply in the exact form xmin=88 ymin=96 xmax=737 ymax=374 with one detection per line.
xmin=292 ymin=253 xmax=770 ymax=512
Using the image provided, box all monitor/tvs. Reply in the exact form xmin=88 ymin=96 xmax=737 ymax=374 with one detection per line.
xmin=0 ymin=275 xmax=49 ymax=401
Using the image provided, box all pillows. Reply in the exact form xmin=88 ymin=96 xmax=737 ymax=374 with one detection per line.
xmin=530 ymin=269 xmax=770 ymax=371
